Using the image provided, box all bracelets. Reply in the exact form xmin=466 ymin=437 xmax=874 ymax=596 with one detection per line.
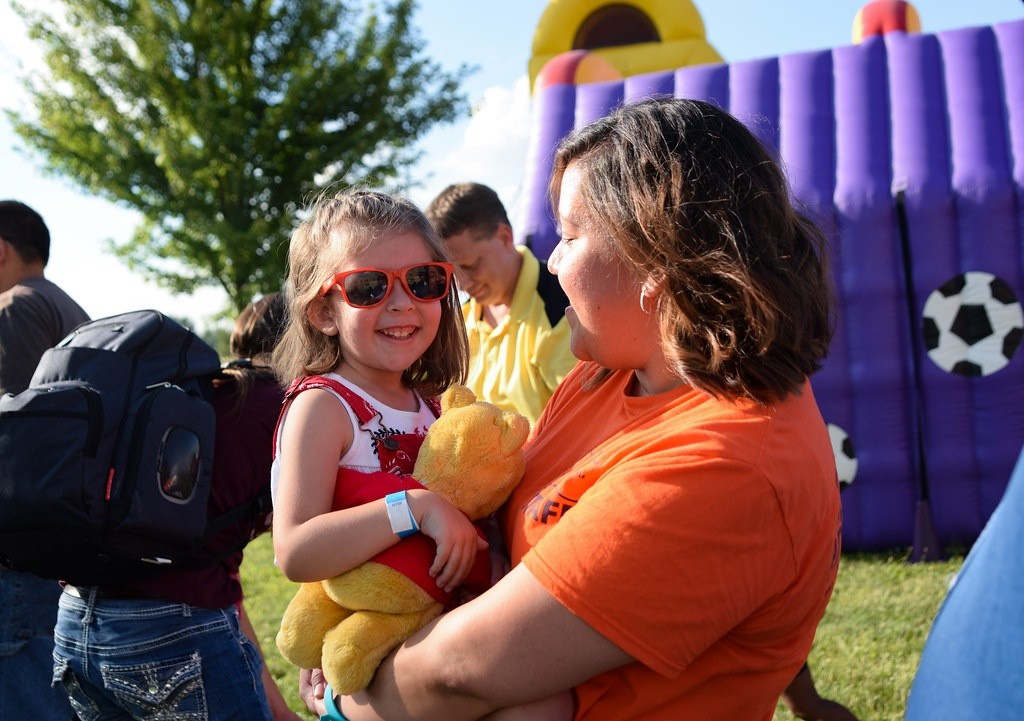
xmin=320 ymin=684 xmax=349 ymax=721
xmin=385 ymin=490 xmax=420 ymax=539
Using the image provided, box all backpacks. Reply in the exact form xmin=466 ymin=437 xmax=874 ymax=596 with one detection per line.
xmin=0 ymin=313 xmax=272 ymax=586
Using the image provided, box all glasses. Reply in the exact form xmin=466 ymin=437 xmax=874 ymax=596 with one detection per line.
xmin=318 ymin=262 xmax=453 ymax=308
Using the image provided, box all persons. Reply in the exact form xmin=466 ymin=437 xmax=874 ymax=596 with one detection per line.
xmin=51 ymin=292 xmax=292 ymax=721
xmin=0 ymin=200 xmax=93 ymax=721
xmin=272 ymin=96 xmax=857 ymax=721
